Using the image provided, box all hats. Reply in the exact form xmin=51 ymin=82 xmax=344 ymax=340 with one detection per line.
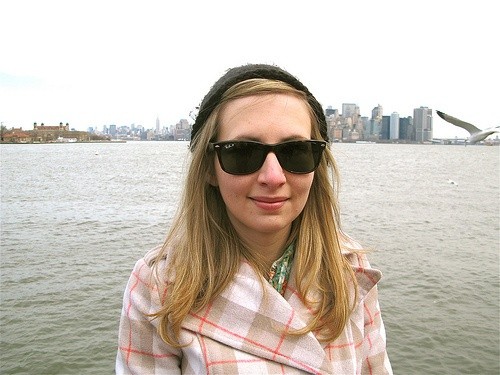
xmin=186 ymin=63 xmax=329 ymax=150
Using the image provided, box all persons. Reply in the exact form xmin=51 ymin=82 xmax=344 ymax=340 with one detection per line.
xmin=115 ymin=64 xmax=395 ymax=375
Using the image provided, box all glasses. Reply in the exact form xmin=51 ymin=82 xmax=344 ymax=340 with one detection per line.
xmin=208 ymin=138 xmax=327 ymax=176
